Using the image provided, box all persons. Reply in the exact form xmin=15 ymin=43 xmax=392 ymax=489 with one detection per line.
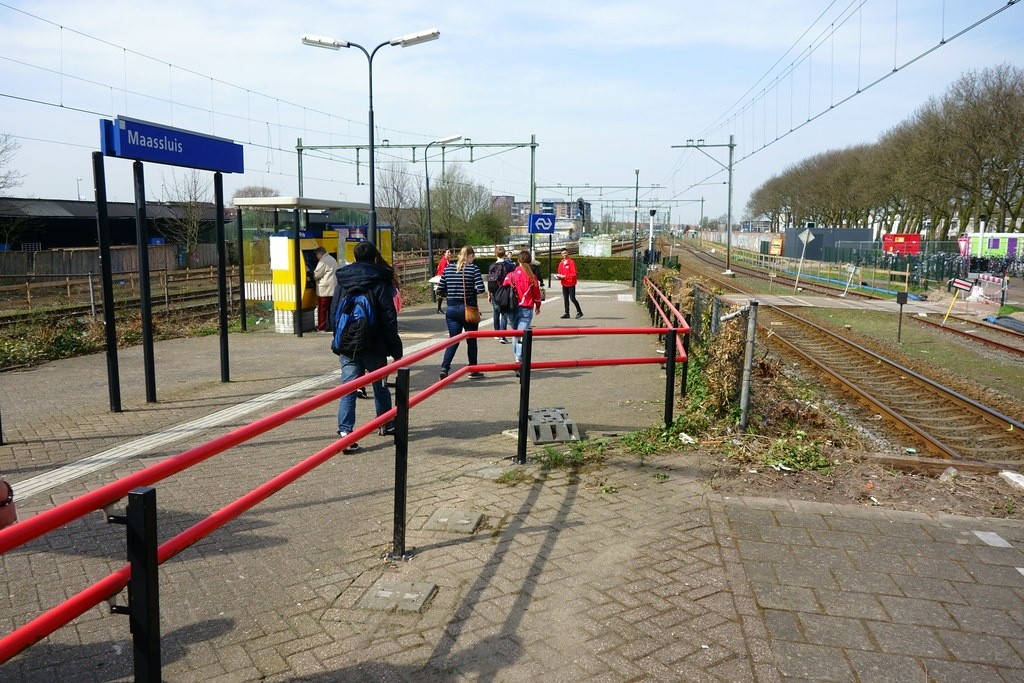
xmin=440 ymin=246 xmax=485 ymax=380
xmin=503 ymin=251 xmax=541 ymax=377
xmin=508 ymin=252 xmax=513 ymax=263
xmin=555 ymin=248 xmax=583 ymax=318
xmin=329 ymin=243 xmax=401 ymax=453
xmin=488 ymin=246 xmax=515 ymax=343
xmin=436 ymin=249 xmax=451 ymax=314
xmin=313 ymin=247 xmax=338 ymax=334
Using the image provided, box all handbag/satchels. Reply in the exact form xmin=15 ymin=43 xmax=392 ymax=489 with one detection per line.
xmin=508 ymin=272 xmax=518 ymax=314
xmin=465 ymin=305 xmax=481 ymax=322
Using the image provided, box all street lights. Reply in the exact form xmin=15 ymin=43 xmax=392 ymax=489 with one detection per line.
xmin=630 ymin=168 xmax=640 ymax=289
xmin=1003 ymin=169 xmax=1009 ymax=233
xmin=424 ymin=135 xmax=463 ymax=301
xmin=301 ymin=28 xmax=445 ymax=244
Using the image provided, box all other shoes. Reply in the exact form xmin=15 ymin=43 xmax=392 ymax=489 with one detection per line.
xmin=468 ymin=371 xmax=484 ymax=379
xmin=500 ymin=337 xmax=508 ymax=344
xmin=561 ymin=313 xmax=570 ymax=319
xmin=495 ymin=337 xmax=499 ymax=340
xmin=575 ymin=311 xmax=583 ymax=318
xmin=516 ymin=370 xmax=521 ymax=377
xmin=356 ymin=387 xmax=367 ymax=398
xmin=323 ymin=327 xmax=333 ymax=334
xmin=439 ymin=369 xmax=448 ymax=381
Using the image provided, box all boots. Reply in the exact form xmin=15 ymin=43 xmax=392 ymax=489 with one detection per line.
xmin=436 ymin=297 xmax=446 ymax=314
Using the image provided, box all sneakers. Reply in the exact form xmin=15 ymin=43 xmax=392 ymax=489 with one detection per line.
xmin=341 ymin=442 xmax=359 ymax=455
xmin=378 ymin=425 xmax=395 ymax=436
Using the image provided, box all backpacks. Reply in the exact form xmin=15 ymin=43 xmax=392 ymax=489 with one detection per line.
xmin=488 ymin=260 xmax=507 ymax=294
xmin=331 ymin=282 xmax=376 ymax=358
